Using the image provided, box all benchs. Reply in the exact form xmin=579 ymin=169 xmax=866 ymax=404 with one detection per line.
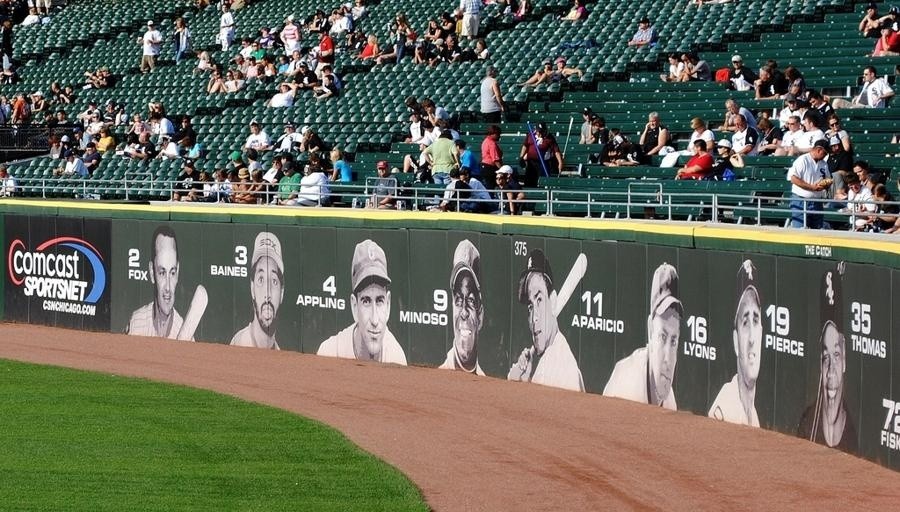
xmin=0 ymin=0 xmax=900 ymax=230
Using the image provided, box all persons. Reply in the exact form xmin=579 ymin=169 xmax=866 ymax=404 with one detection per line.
xmin=700 ymin=256 xmax=766 ymax=432
xmin=503 ymin=248 xmax=588 ymax=397
xmin=794 ymin=270 xmax=863 ymax=459
xmin=432 ymin=237 xmax=489 ymax=379
xmin=227 ymin=230 xmax=288 ymax=351
xmin=599 ymin=259 xmax=686 ymax=414
xmin=0 ymin=1 xmax=895 ymax=235
xmin=119 ymin=225 xmax=197 ymax=346
xmin=310 ymin=238 xmax=411 ymax=368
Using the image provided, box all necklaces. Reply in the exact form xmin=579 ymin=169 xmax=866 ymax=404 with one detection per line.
xmin=451 ymin=337 xmax=479 ymax=374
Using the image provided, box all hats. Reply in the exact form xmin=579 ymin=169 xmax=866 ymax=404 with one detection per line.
xmin=815 ymin=140 xmax=832 ymax=153
xmin=300 ymin=63 xmax=307 ymax=67
xmin=252 ymin=232 xmax=284 ymax=276
xmin=377 ymin=161 xmax=388 ymax=168
xmin=352 ymin=240 xmax=391 ymax=292
xmin=714 ymin=139 xmax=731 ymax=148
xmin=820 ymin=268 xmax=843 ymax=339
xmin=181 ymin=160 xmax=197 ymax=167
xmin=830 ymin=137 xmax=840 ymax=146
xmin=581 ymin=107 xmax=592 ymax=114
xmin=450 ymin=169 xmax=459 ymax=177
xmin=61 ymin=100 xmax=169 ymax=157
xmin=613 ymin=135 xmax=628 ymax=149
xmin=406 ymin=107 xmax=418 ymax=117
xmin=866 ymin=2 xmax=900 ymax=28
xmin=542 ymin=57 xmax=567 ymax=65
xmin=536 ymin=121 xmax=546 ymax=131
xmin=787 ymin=95 xmax=796 ymax=101
xmin=99 ymin=67 xmax=109 ymax=71
xmin=518 ymin=249 xmax=554 ymax=302
xmin=231 ymin=121 xmax=343 ymax=179
xmin=31 ymin=91 xmax=44 ymax=96
xmin=147 ymin=20 xmax=154 ymax=25
xmin=637 ymin=18 xmax=649 ymax=23
xmin=733 ymin=259 xmax=762 ymax=325
xmin=650 ymin=263 xmax=685 ymax=319
xmin=495 ymin=165 xmax=513 ymax=174
xmin=732 ymin=55 xmax=742 ymax=61
xmin=450 ymin=240 xmax=483 ymax=307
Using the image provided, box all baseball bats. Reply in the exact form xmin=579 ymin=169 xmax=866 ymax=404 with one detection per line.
xmin=526 ymin=250 xmax=588 ymax=361
xmin=178 ymin=285 xmax=207 ymax=340
